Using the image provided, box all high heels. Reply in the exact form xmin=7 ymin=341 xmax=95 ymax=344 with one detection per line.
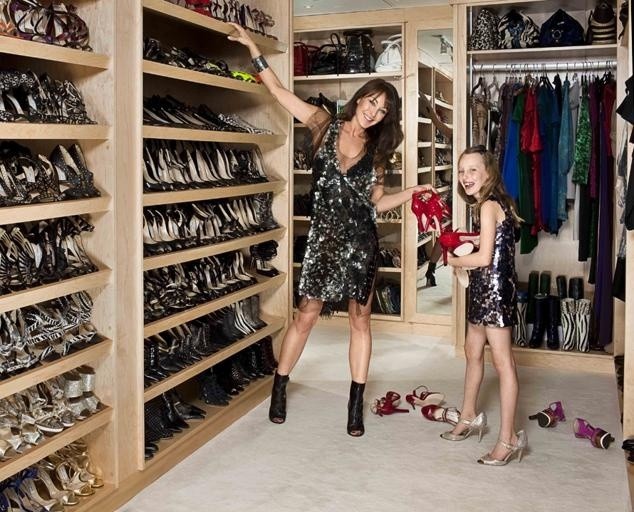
xmin=370 ymin=385 xmax=486 ymax=442
xmin=573 ymin=418 xmax=614 ymax=449
xmin=143 ymin=192 xmax=279 ymax=256
xmin=439 ymin=232 xmax=479 ymax=266
xmin=143 ymin=95 xmax=273 ymax=134
xmin=144 ymin=240 xmax=289 ymax=464
xmin=477 ymin=430 xmax=528 ymax=466
xmin=412 ymin=189 xmax=480 ymax=236
xmin=143 ymin=139 xmax=269 ymax=193
xmin=529 ymin=402 xmax=565 ymax=428
xmin=165 ymin=0 xmax=275 ymax=36
xmin=426 ymin=261 xmax=436 ymax=286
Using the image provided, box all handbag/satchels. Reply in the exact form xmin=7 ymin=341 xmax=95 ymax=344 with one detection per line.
xmin=468 ymin=0 xmax=617 ymax=51
xmin=294 ymin=29 xmax=402 ymax=76
xmin=376 ymin=278 xmax=401 ymax=314
xmin=293 ymin=151 xmax=311 ymax=169
xmin=379 ymin=246 xmax=400 ymax=267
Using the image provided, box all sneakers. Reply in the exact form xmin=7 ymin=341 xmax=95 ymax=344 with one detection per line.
xmin=143 ymin=38 xmax=257 ymax=83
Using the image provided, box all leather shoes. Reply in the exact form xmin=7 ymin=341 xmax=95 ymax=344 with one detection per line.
xmin=0 ymin=70 xmax=97 ymax=124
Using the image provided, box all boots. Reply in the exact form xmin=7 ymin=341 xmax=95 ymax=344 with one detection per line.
xmin=348 ymin=381 xmax=365 ymax=436
xmin=514 ymin=272 xmax=603 ymax=352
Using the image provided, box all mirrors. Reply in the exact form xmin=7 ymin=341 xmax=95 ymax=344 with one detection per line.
xmin=412 ymin=23 xmax=458 ymax=322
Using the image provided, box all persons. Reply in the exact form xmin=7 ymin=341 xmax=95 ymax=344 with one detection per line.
xmin=418 ymin=89 xmax=452 ymax=286
xmin=439 ymin=146 xmax=525 ymax=465
xmin=226 ymin=22 xmax=403 ymax=437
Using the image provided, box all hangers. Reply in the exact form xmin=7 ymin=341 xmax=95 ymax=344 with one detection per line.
xmin=467 ymin=58 xmax=615 ymax=104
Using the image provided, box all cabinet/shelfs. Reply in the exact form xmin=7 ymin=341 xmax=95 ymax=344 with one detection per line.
xmin=451 ymin=47 xmax=615 ymax=377
xmin=416 ymin=45 xmax=455 ymax=284
xmin=139 ymin=0 xmax=298 ymax=493
xmin=610 ymin=0 xmax=634 ymax=512
xmin=291 ymin=7 xmax=408 ymax=323
xmin=0 ymin=0 xmax=140 ymax=512
xmin=457 ymin=0 xmax=619 ymax=55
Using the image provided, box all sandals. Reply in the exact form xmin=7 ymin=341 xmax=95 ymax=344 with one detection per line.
xmin=0 ymin=438 xmax=104 ymax=512
xmin=0 ymin=216 xmax=99 ymax=295
xmin=0 ymin=0 xmax=93 ymax=52
xmin=0 ymin=143 xmax=101 ymax=206
xmin=0 ymin=365 xmax=102 ymax=461
xmin=0 ymin=290 xmax=103 ymax=381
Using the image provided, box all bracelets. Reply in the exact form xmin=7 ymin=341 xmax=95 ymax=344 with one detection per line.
xmin=250 ymin=55 xmax=268 ymax=73
